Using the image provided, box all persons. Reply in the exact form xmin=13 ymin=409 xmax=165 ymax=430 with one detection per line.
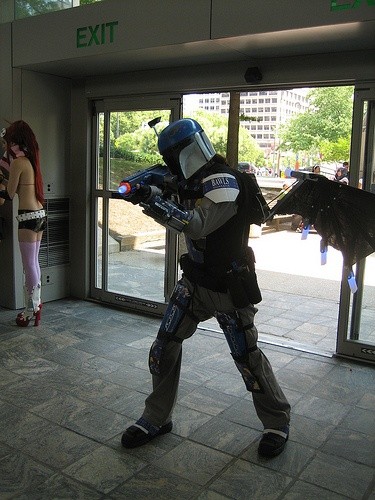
xmin=121 ymin=118 xmax=290 ymax=458
xmin=334 ymin=162 xmax=350 ymax=185
xmin=279 ymin=184 xmax=290 ymax=200
xmin=0 ymin=121 xmax=48 ymax=327
xmin=312 ymin=165 xmax=320 ymax=174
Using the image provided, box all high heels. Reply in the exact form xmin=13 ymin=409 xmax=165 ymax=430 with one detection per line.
xmin=15 ymin=303 xmax=42 ymax=327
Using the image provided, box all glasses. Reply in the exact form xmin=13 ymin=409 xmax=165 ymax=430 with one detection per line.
xmin=0 ymin=127 xmax=8 ymax=144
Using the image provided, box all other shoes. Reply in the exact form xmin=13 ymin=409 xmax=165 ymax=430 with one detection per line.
xmin=258 ymin=429 xmax=289 ymax=460
xmin=121 ymin=415 xmax=173 ymax=448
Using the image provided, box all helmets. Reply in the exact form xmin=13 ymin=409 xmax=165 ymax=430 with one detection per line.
xmin=158 ymin=118 xmax=217 ymax=180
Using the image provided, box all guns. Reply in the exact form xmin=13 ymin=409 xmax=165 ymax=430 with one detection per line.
xmin=117 ymin=164 xmax=174 ymax=195
